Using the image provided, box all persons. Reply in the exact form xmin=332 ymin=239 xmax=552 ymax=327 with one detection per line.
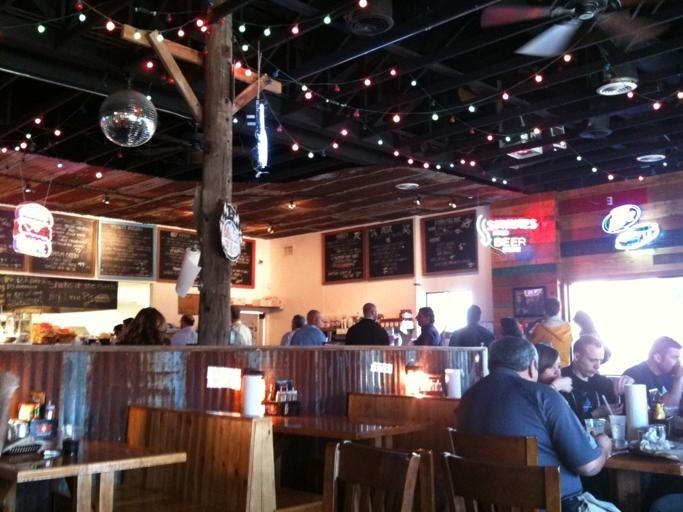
xmin=453 ymin=336 xmax=683 ymax=512
xmin=114 ymin=302 xmax=252 ymax=412
xmin=279 ymin=301 xmax=611 ymax=368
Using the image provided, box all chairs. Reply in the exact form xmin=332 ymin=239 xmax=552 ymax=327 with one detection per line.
xmin=446 ymin=426 xmax=539 ymax=467
xmin=443 ymin=451 xmax=562 ymax=512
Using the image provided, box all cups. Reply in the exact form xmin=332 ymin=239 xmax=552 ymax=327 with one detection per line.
xmin=584 ymin=417 xmax=607 ymax=435
xmin=650 ymin=423 xmax=666 ymax=446
xmin=608 ymin=415 xmax=627 ymax=447
xmin=61 ymin=422 xmax=82 ymax=460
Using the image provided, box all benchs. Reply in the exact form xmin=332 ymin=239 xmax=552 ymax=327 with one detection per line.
xmin=57 ymin=392 xmax=460 ymax=512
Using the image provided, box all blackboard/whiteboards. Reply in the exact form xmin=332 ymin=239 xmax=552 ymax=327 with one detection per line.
xmin=0 ymin=274 xmax=118 ymax=316
xmin=156 ymin=226 xmax=256 ymax=292
xmin=0 ymin=203 xmax=98 ymax=285
xmin=319 ymin=215 xmax=421 ymax=285
xmin=99 ymin=219 xmax=156 ymax=282
xmin=419 ymin=209 xmax=480 ymax=277
